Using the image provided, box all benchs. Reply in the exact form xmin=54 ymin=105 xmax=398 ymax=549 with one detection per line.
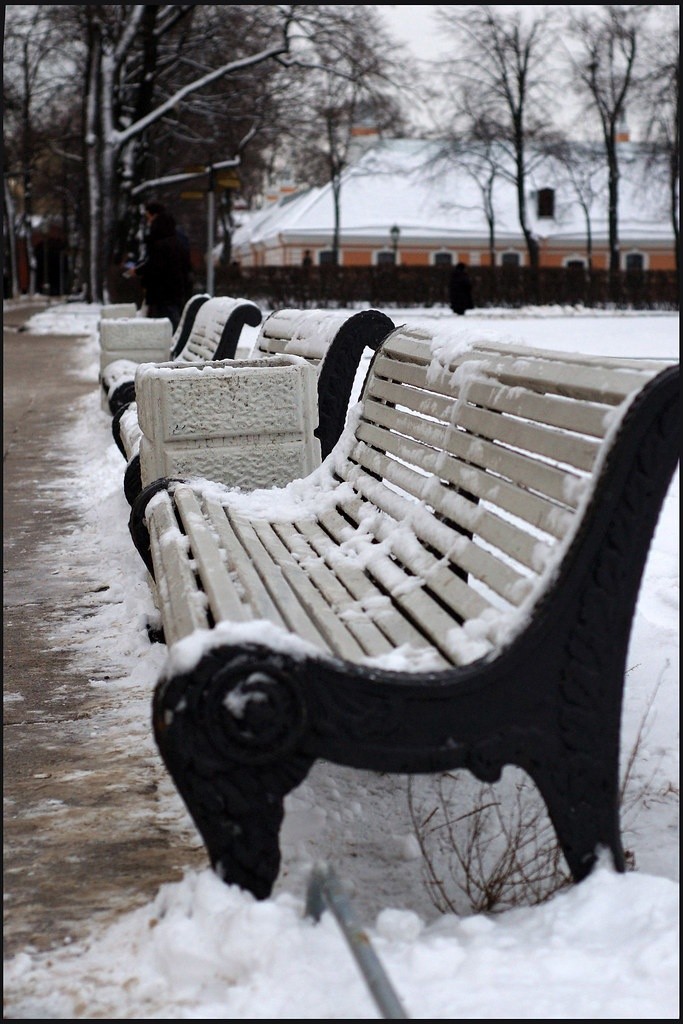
xmin=104 ymin=292 xmax=683 ymax=902
